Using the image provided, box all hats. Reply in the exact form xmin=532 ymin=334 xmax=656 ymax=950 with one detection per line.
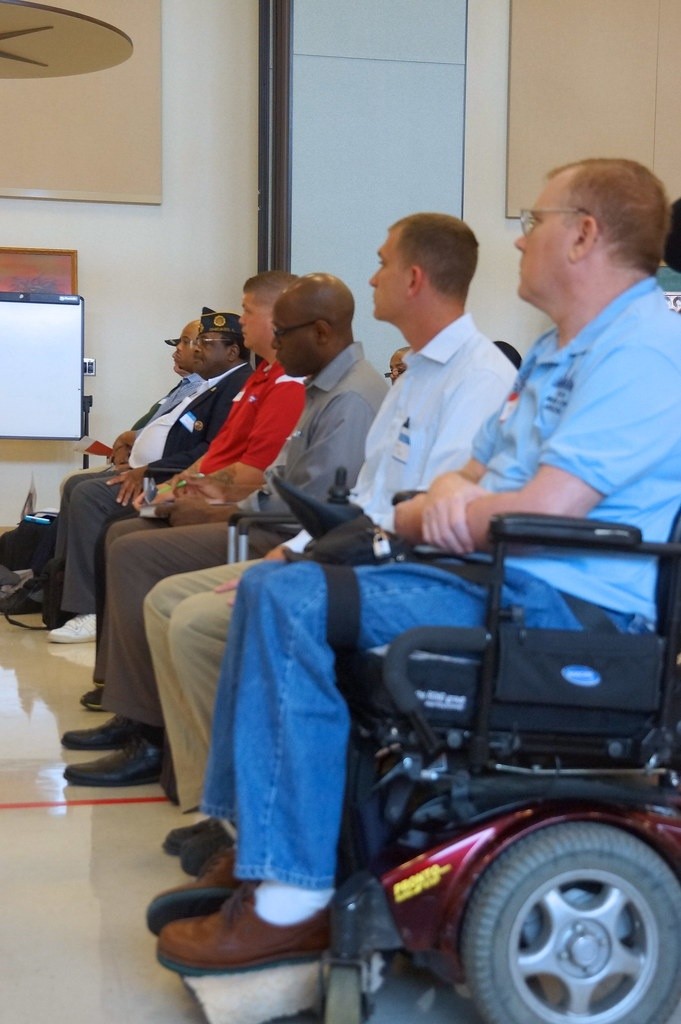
xmin=198 ymin=307 xmax=241 ymax=335
xmin=495 ymin=341 xmax=522 ymax=369
xmin=165 ymin=339 xmax=180 ymax=346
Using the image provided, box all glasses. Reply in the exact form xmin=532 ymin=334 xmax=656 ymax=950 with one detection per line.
xmin=190 ymin=338 xmax=231 ymax=346
xmin=384 ymin=369 xmax=403 ymax=377
xmin=520 ymin=208 xmax=591 ymax=237
xmin=273 ymin=322 xmax=315 ymax=337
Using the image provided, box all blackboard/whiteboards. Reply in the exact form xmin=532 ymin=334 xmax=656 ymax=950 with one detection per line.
xmin=0 ymin=291 xmax=84 ymax=441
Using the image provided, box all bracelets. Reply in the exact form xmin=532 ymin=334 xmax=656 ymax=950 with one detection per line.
xmin=114 ymin=444 xmax=125 ymax=453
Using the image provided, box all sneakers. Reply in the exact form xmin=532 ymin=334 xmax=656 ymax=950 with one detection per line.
xmin=147 ymin=845 xmax=242 ymax=937
xmin=157 ymin=882 xmax=331 ymax=976
xmin=81 ymin=682 xmax=104 ymax=709
xmin=50 ymin=614 xmax=96 ymax=643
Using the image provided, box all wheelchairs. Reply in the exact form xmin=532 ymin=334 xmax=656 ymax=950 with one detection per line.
xmin=146 ymin=510 xmax=681 ymax=1024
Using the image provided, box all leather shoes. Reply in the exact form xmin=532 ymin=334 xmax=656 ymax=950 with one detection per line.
xmin=181 ymin=819 xmax=237 ymax=876
xmin=64 ymin=736 xmax=164 ymax=786
xmin=163 ymin=817 xmax=219 ymax=855
xmin=62 ymin=713 xmax=133 ymax=749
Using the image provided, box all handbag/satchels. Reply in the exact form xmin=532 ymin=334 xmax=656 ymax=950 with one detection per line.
xmin=314 ymin=514 xmax=407 ymax=564
xmin=5 ymin=557 xmax=76 ymax=630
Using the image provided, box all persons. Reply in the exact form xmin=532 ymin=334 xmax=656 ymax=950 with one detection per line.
xmin=389 ymin=346 xmax=416 ymax=386
xmin=59 ymin=272 xmax=390 ymax=789
xmin=0 ymin=270 xmax=310 ymax=715
xmin=142 ymin=214 xmax=519 ymax=877
xmin=140 ymin=153 xmax=681 ymax=976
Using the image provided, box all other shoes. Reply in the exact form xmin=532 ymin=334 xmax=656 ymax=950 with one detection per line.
xmin=160 ymin=760 xmax=179 ymax=805
